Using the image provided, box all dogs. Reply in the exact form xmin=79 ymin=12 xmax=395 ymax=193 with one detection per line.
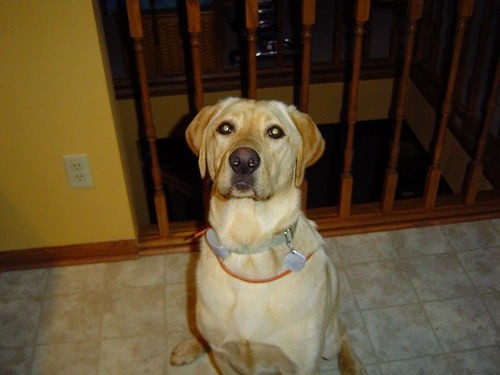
xmin=170 ymin=96 xmax=367 ymax=375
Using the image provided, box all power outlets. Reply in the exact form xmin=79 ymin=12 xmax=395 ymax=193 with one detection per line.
xmin=61 ymin=153 xmax=93 ymax=188
